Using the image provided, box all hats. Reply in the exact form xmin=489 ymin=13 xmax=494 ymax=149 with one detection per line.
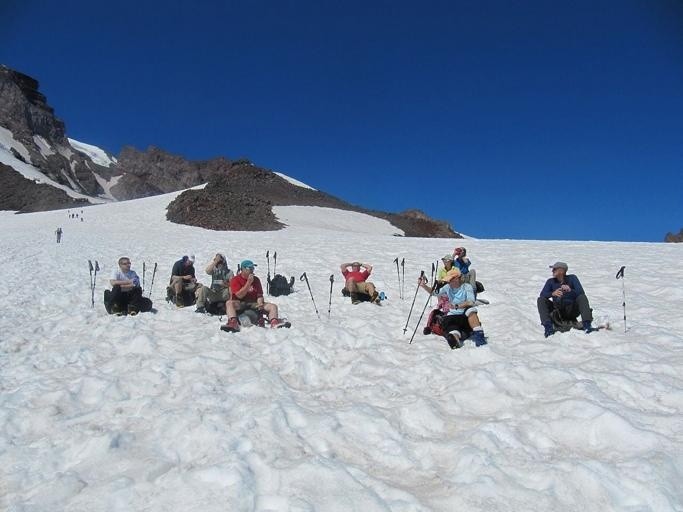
xmin=241 ymin=261 xmax=258 ymax=268
xmin=549 ymin=262 xmax=568 ymax=270
xmin=440 ymin=270 xmax=461 ymax=282
xmin=441 ymin=255 xmax=453 ymax=261
xmin=188 ymin=253 xmax=196 ymax=262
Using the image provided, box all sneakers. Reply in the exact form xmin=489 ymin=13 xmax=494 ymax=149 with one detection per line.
xmin=541 ymin=320 xmax=555 ymax=338
xmin=195 ymin=308 xmax=205 ymax=314
xmin=113 ymin=304 xmax=122 ymax=316
xmin=582 ymin=320 xmax=592 ymax=333
xmin=371 ymin=291 xmax=380 ymax=304
xmin=473 ymin=330 xmax=487 ymax=346
xmin=352 ymin=298 xmax=359 ymax=305
xmin=128 ymin=305 xmax=137 ymax=316
xmin=270 ymin=318 xmax=291 ymax=329
xmin=447 ymin=334 xmax=462 ymax=349
xmin=221 ymin=317 xmax=241 ymax=333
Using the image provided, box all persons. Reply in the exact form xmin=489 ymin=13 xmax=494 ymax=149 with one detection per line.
xmin=438 ymin=254 xmax=461 ymax=280
xmin=110 ymin=256 xmax=142 ymax=316
xmin=418 ymin=269 xmax=488 ymax=350
xmin=195 ymin=253 xmax=234 ymax=313
xmin=220 ymin=260 xmax=291 ymax=333
xmin=56 ymin=227 xmax=63 ymax=243
xmin=340 ymin=262 xmax=381 ymax=304
xmin=451 ymin=247 xmax=477 ymax=293
xmin=169 ymin=255 xmax=202 ymax=307
xmin=537 ymin=262 xmax=599 ymax=338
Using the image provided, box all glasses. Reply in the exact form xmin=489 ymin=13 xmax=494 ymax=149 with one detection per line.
xmin=121 ymin=261 xmax=131 ymax=266
xmin=443 ymin=259 xmax=451 ymax=262
xmin=245 ymin=266 xmax=254 ymax=270
xmin=353 ymin=265 xmax=360 ymax=267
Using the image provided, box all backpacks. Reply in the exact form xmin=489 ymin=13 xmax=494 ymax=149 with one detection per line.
xmin=268 ymin=274 xmax=295 ymax=297
xmin=204 ymin=299 xmax=227 ymax=316
xmin=433 ymin=279 xmax=449 ymax=294
xmin=341 ymin=287 xmax=378 ymax=302
xmin=548 ymin=309 xmax=584 ymax=332
xmin=166 ymin=286 xmax=196 ymax=305
xmin=427 ymin=308 xmax=482 ymax=336
xmin=104 ymin=289 xmax=153 ymax=314
